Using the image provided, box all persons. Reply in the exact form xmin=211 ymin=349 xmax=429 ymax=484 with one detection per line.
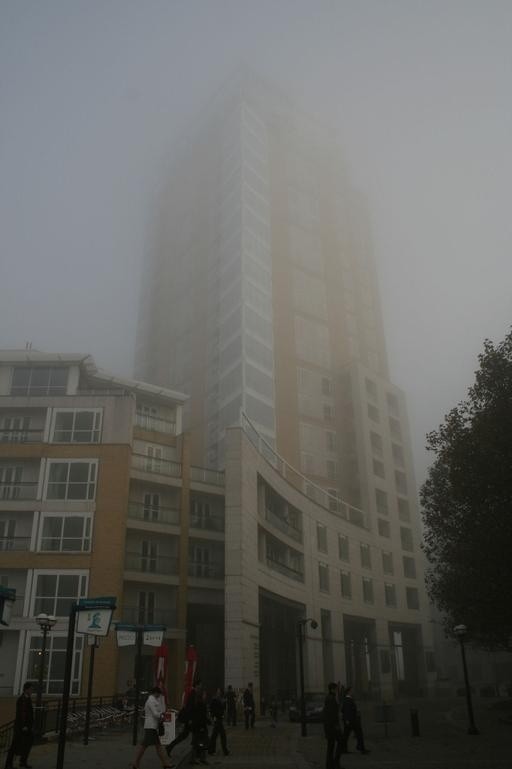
xmin=341 ymin=687 xmax=371 ymax=753
xmin=130 ymin=687 xmax=177 ymax=769
xmin=224 ymin=685 xmax=237 ymax=727
xmin=6 ymin=682 xmax=36 ymax=768
xmin=208 ymin=688 xmax=231 ymax=755
xmin=323 ymin=683 xmax=345 ymax=769
xmin=166 ymin=681 xmax=203 ymax=763
xmin=195 ymin=691 xmax=209 ymax=756
xmin=243 ymin=682 xmax=256 ymax=728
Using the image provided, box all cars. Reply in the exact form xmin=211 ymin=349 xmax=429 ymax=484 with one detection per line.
xmin=286 ymin=689 xmax=327 ymax=723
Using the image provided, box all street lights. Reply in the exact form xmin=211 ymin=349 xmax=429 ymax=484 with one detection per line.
xmin=82 ymin=630 xmax=99 ymax=746
xmin=450 ymin=622 xmax=483 ymax=736
xmin=295 ymin=614 xmax=318 ymax=737
xmin=32 ymin=613 xmax=58 ymax=751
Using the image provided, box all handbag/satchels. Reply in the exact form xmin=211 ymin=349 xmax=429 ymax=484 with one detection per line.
xmin=157 ymin=719 xmax=166 ymax=737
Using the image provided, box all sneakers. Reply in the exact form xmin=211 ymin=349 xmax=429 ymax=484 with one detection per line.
xmin=163 ymin=765 xmax=175 ymax=769
xmin=166 ymin=746 xmax=172 ymax=757
xmin=195 ymin=744 xmax=232 ymax=764
xmin=132 ymin=765 xmax=137 ymax=769
xmin=339 ymin=745 xmax=371 ymax=755
